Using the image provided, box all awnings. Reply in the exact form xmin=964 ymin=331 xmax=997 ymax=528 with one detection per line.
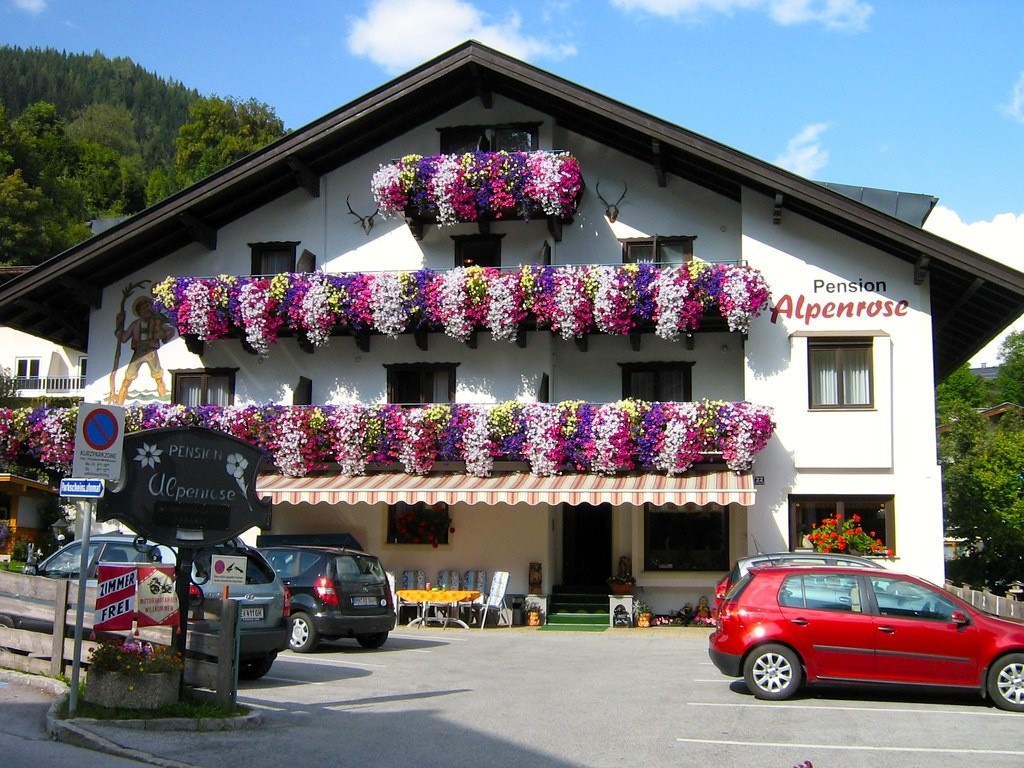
xmin=255 ymin=470 xmax=757 ymax=506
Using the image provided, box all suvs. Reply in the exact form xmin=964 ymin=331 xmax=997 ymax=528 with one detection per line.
xmin=23 ymin=530 xmax=296 ymax=681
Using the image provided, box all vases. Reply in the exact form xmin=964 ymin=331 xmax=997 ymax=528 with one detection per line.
xmin=849 ymin=546 xmax=867 ymax=556
xmin=802 ymin=534 xmax=815 ymax=549
xmin=609 ymin=582 xmax=633 ymax=594
xmin=84 ymin=667 xmax=180 ymax=712
xmin=637 ymin=612 xmax=651 ymax=627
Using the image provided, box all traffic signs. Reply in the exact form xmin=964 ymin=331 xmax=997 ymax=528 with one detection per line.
xmin=60 ymin=478 xmax=105 ymax=497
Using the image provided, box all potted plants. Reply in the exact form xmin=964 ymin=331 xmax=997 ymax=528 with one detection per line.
xmin=521 ymin=601 xmax=545 ymax=626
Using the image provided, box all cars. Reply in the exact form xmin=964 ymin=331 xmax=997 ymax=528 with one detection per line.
xmin=708 ymin=534 xmax=1024 ymax=712
xmin=254 ymin=545 xmax=396 ymax=653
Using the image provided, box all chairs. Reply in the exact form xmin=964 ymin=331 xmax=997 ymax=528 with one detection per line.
xmin=427 ymin=570 xmax=459 ymax=626
xmin=385 ymin=570 xmax=398 ymax=628
xmin=460 ymin=570 xmax=487 ymax=628
xmin=851 ymin=589 xmax=860 ymax=612
xmin=399 ymin=570 xmax=426 ymax=626
xmin=468 ymin=570 xmax=511 ymax=629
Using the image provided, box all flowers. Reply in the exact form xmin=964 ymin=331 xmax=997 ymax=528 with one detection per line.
xmin=808 ymin=511 xmax=848 ymax=554
xmin=606 ymin=570 xmax=636 ymax=585
xmin=372 ymin=149 xmax=584 ymax=232
xmin=1 ymin=397 xmax=777 ymax=479
xmin=86 ymin=631 xmax=185 ymax=693
xmin=151 ymin=256 xmax=774 ymax=361
xmin=633 ymin=599 xmax=651 ymax=620
xmin=842 ymin=514 xmax=893 ymax=556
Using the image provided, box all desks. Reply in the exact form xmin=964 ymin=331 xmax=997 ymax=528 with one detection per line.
xmin=395 ymin=590 xmax=481 ymax=629
